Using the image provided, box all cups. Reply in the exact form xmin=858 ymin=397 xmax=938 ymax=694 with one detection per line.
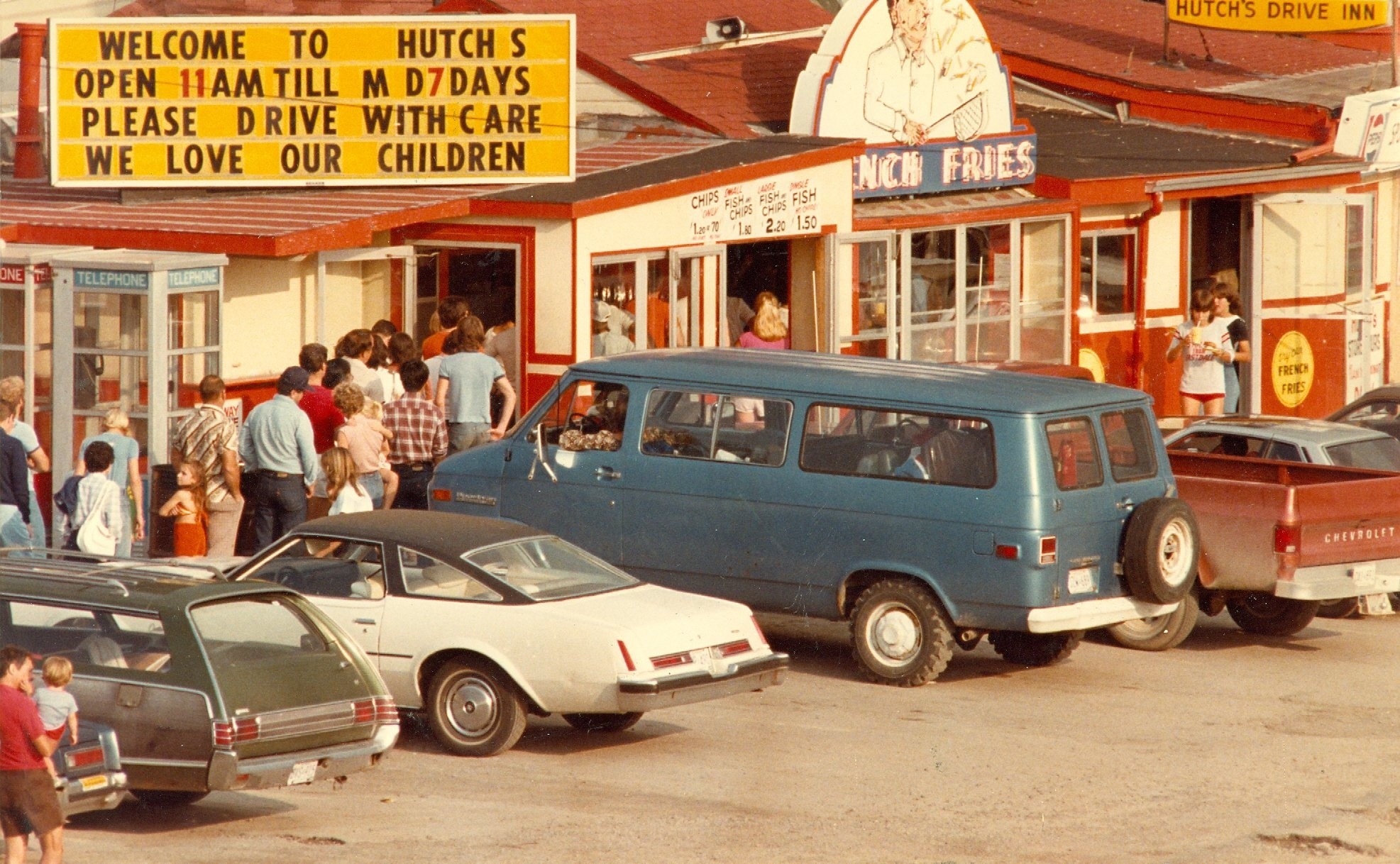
xmin=1191 ymin=327 xmax=1202 ymax=345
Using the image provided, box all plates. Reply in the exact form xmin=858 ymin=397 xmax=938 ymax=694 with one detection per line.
xmin=1203 ymin=348 xmax=1220 ymax=351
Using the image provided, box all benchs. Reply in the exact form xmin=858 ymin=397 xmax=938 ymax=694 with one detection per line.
xmin=766 ymin=434 xmax=864 ymax=472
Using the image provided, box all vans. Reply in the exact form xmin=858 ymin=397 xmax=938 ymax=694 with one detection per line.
xmin=426 ymin=344 xmax=1203 ymax=689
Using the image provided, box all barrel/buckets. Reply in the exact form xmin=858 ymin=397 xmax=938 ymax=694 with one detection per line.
xmin=234 ymin=472 xmax=283 ymax=557
xmin=146 ymin=464 xmax=181 ymax=559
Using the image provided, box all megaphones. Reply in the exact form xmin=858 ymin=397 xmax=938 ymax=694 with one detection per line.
xmin=705 ymin=16 xmax=745 ymax=42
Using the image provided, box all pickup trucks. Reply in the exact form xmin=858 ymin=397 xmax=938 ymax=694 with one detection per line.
xmin=1054 ymin=444 xmax=1400 ymax=654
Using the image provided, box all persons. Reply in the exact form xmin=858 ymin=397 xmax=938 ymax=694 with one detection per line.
xmin=0 ymin=292 xmax=797 ymax=565
xmin=1165 ymin=283 xmax=1251 ymax=421
xmin=0 ymin=645 xmax=81 ymax=864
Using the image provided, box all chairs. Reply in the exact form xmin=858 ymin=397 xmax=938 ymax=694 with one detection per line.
xmin=349 ymin=565 xmax=468 ymax=597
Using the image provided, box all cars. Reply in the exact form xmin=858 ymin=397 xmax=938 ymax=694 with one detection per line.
xmin=43 ymin=720 xmax=128 ymax=827
xmin=1325 ymin=381 xmax=1400 ymax=441
xmin=0 ymin=544 xmax=401 ymax=805
xmin=98 ymin=508 xmax=792 ymax=757
xmin=1163 ymin=412 xmax=1400 ymax=474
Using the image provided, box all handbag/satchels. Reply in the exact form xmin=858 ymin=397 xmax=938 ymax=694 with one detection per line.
xmin=75 ymin=511 xmax=119 ymax=563
